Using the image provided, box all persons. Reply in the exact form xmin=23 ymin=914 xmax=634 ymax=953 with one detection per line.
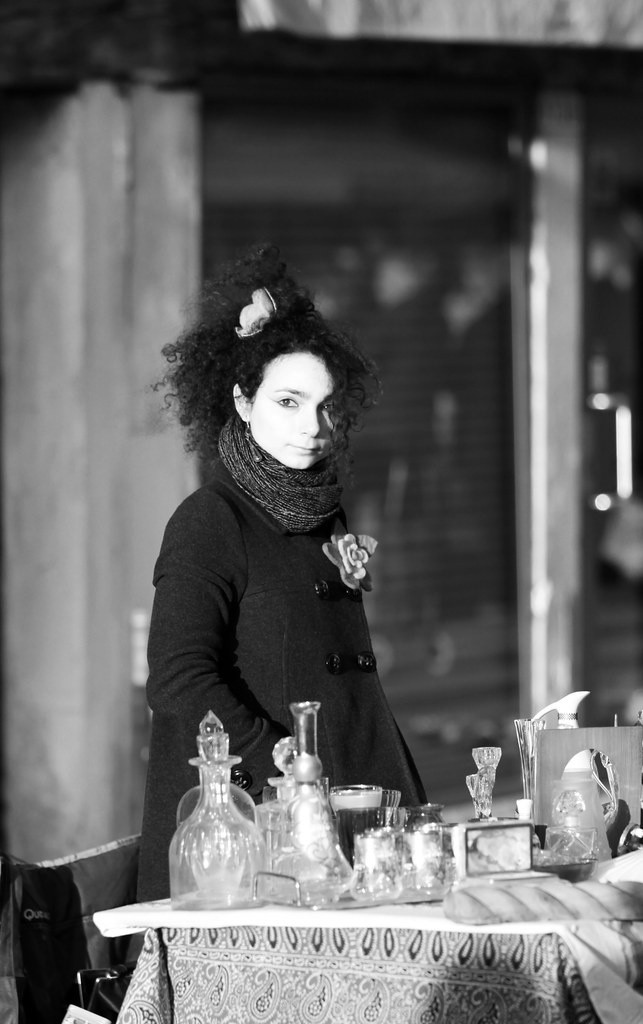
xmin=124 ymin=271 xmax=430 ymax=959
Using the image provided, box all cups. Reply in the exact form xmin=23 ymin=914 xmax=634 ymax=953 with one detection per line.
xmin=329 ymin=784 xmax=384 ymax=869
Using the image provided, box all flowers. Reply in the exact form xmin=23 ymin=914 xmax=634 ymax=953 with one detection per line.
xmin=321 ymin=517 xmax=378 ymax=592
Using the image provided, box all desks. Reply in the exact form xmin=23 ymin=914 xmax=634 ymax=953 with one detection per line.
xmin=93 ymin=900 xmax=643 ymax=1024
